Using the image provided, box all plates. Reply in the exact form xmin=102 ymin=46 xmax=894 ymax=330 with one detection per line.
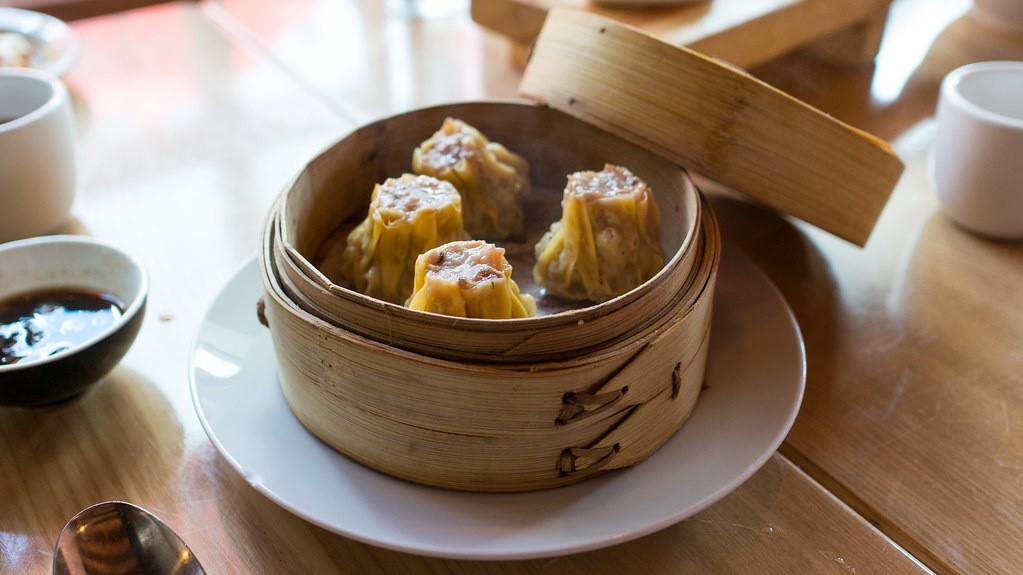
xmin=191 ymin=203 xmax=809 ymax=558
xmin=1 ymin=4 xmax=79 ymax=80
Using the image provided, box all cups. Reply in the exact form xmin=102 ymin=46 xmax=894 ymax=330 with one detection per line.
xmin=931 ymin=54 xmax=1023 ymax=243
xmin=1 ymin=65 xmax=87 ymax=243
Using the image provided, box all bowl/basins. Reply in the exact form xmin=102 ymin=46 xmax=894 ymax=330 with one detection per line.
xmin=0 ymin=231 xmax=152 ymax=416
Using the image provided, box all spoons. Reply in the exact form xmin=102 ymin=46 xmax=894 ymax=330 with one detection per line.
xmin=50 ymin=498 xmax=206 ymax=574
